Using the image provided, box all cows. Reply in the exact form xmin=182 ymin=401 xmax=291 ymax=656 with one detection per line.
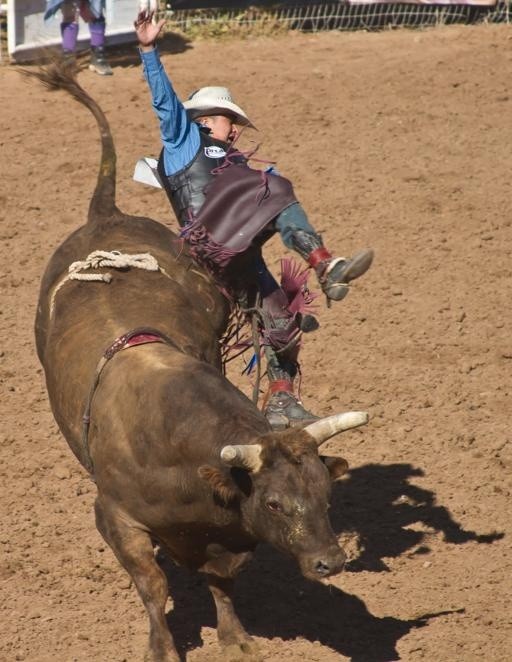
xmin=14 ymin=44 xmax=370 ymax=661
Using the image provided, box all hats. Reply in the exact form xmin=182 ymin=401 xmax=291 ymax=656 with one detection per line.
xmin=181 ymin=86 xmax=260 ymax=133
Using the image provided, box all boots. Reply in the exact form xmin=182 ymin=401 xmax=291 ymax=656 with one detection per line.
xmin=292 ymin=229 xmax=375 ymax=301
xmin=258 ymin=318 xmax=321 ymax=432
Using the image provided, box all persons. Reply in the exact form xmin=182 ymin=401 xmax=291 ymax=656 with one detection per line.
xmin=44 ymin=1 xmax=116 ymax=76
xmin=134 ymin=0 xmax=375 ymax=430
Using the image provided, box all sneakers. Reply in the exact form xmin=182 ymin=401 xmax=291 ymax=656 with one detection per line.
xmin=89 ymin=55 xmax=114 ymax=78
